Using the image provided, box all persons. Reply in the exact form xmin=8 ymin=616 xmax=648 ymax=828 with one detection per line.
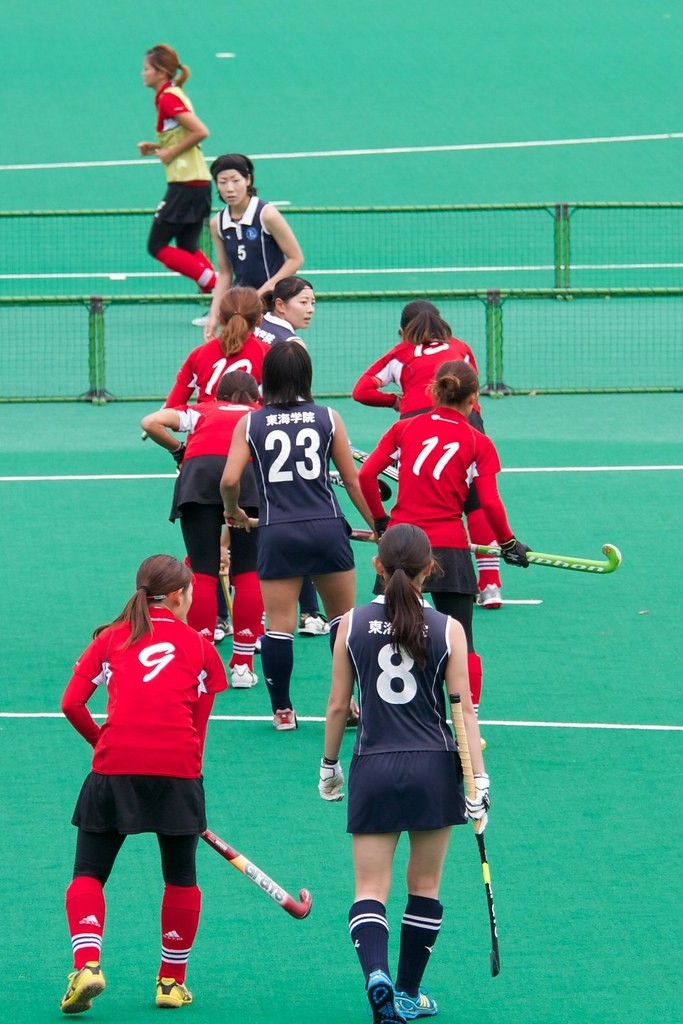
xmin=164 ymin=287 xmax=265 ymax=651
xmin=320 ymin=525 xmax=490 ymax=1024
xmin=141 ymin=371 xmax=266 ymax=690
xmin=353 ymin=300 xmax=502 ymax=608
xmin=254 ymin=276 xmax=330 ymax=634
xmin=358 ymin=361 xmax=534 ymax=749
xmin=137 ymin=47 xmax=218 ymax=293
xmin=220 ymin=341 xmax=376 ymax=730
xmin=204 ymin=153 xmax=304 ymax=342
xmin=60 ymin=555 xmax=227 ymax=1013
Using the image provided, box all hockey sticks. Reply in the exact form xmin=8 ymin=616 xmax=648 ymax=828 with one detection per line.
xmin=199 ymin=829 xmax=313 ymax=919
xmin=329 ymin=473 xmax=393 ymax=503
xmin=449 ymin=691 xmax=501 ymax=978
xmin=470 ymin=543 xmax=622 ymax=574
xmin=351 ymin=445 xmax=400 ymax=481
xmin=218 ymin=566 xmax=233 ymax=621
xmin=227 ymin=516 xmax=377 ymax=543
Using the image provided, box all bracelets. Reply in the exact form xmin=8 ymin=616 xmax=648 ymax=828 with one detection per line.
xmin=323 ymin=757 xmax=338 ymax=765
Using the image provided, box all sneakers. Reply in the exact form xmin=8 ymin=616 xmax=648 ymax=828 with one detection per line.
xmin=367 ymin=970 xmax=407 ymax=1024
xmin=272 ymin=708 xmax=298 ymax=730
xmin=192 ymin=311 xmax=209 ymax=326
xmin=346 ymin=696 xmax=359 ymax=727
xmin=393 ymin=985 xmax=438 ymax=1019
xmin=231 ymin=663 xmax=258 ymax=687
xmin=475 ymin=583 xmax=502 ymax=609
xmin=255 ymin=624 xmax=266 ymax=651
xmin=60 ymin=961 xmax=105 ymax=1014
xmin=298 ymin=612 xmax=330 ymax=635
xmin=155 ymin=976 xmax=192 ymax=1008
xmin=214 ymin=617 xmax=234 ymax=640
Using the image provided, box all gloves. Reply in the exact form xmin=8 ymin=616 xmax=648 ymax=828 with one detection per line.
xmin=170 ymin=442 xmax=187 ymax=465
xmin=373 ymin=515 xmax=391 ymax=538
xmin=498 ymin=536 xmax=532 ymax=568
xmin=318 ymin=757 xmax=345 ymax=802
xmin=464 ymin=773 xmax=491 ymax=835
